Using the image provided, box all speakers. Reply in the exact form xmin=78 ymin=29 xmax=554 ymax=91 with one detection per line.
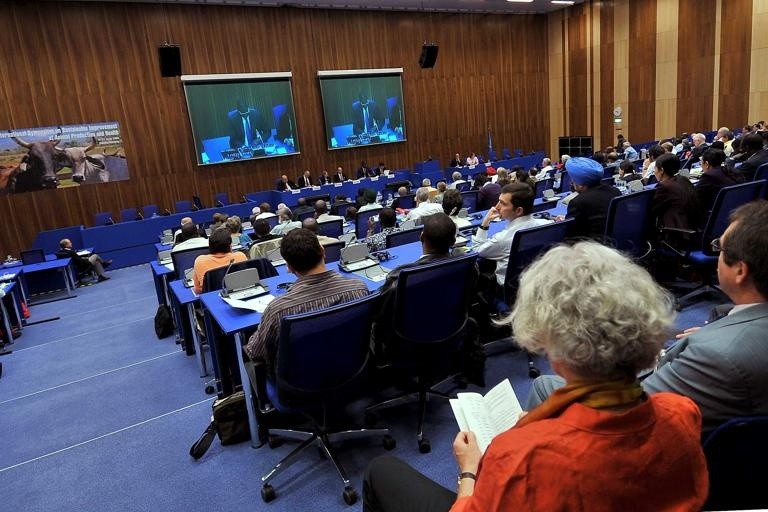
xmin=158 ymin=44 xmax=182 ymax=78
xmin=418 ymin=44 xmax=439 ymax=69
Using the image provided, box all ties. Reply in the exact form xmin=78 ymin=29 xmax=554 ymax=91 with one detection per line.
xmin=364 ymin=108 xmax=369 ymax=132
xmin=244 ymin=117 xmax=252 ymax=145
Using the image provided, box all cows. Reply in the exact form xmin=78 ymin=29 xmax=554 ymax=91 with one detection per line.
xmin=54 ymin=136 xmax=130 ymax=185
xmin=0 ymin=126 xmax=63 ymax=193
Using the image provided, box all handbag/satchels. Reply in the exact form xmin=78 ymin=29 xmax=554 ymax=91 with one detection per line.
xmin=154 ymin=305 xmax=174 ymax=339
xmin=189 ymin=390 xmax=251 ymax=460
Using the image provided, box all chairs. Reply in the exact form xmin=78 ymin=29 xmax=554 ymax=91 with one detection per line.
xmin=242 ymin=292 xmax=399 ymax=504
xmin=599 ymin=187 xmax=660 ymax=270
xmin=91 ymin=128 xmax=445 ymax=292
xmin=661 ymin=179 xmax=766 ymax=313
xmin=483 ymin=217 xmax=577 ymax=378
xmin=444 ymin=130 xmax=766 ymax=259
xmin=366 ymin=254 xmax=490 ymax=457
xmin=754 ymin=163 xmax=767 ymax=179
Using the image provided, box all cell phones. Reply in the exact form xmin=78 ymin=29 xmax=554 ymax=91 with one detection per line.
xmin=369 ymin=217 xmax=374 ymax=224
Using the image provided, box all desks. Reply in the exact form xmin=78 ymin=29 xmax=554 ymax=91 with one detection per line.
xmin=0 ymin=246 xmax=94 ymax=357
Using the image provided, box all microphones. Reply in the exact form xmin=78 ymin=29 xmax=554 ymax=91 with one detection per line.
xmin=182 ymin=267 xmax=193 ymax=284
xmin=630 ymin=170 xmax=655 ymax=194
xmin=221 ymin=258 xmax=235 ymax=297
xmin=398 ymin=218 xmax=410 ymax=228
xmin=278 ymin=221 xmax=290 ymax=237
xmin=678 ymin=154 xmax=694 ymax=175
xmin=265 ymin=246 xmax=281 ymax=262
xmin=543 ymin=179 xmax=549 ymax=202
xmin=317 ymin=177 xmax=323 ymax=187
xmin=338 ymin=236 xmax=355 ymax=270
xmin=108 ymin=196 xmax=248 ymax=224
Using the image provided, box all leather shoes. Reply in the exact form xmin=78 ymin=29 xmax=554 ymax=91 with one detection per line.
xmin=98 ymin=276 xmax=110 ymax=282
xmin=103 ymin=259 xmax=112 ymax=267
xmin=2 ymin=331 xmax=21 ymax=344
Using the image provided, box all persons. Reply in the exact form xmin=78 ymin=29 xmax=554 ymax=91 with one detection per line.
xmin=226 ymin=97 xmax=271 ymax=149
xmin=275 ymin=159 xmax=393 ymax=192
xmin=355 ymin=235 xmax=708 ymax=512
xmin=350 ymin=91 xmax=384 ymax=137
xmin=389 ymin=99 xmax=402 ymax=135
xmin=451 ymin=152 xmax=480 ymax=166
xmin=280 ymin=110 xmax=294 ymax=146
xmin=58 ymin=238 xmax=114 ymax=283
xmin=173 ymin=171 xmax=481 ymax=447
xmin=471 ymin=126 xmax=767 ymax=443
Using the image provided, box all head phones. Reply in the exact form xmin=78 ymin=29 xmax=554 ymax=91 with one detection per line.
xmin=277 ymin=283 xmax=294 ymax=292
xmin=371 ymin=251 xmax=391 ymax=262
xmin=460 ymin=227 xmax=477 ymax=236
xmin=473 ymin=213 xmax=483 ymax=220
xmin=532 ymin=212 xmax=551 ymax=219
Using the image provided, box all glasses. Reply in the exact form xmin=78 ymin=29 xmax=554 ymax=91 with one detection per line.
xmin=709 ymin=238 xmax=727 ymax=254
xmin=568 ymin=177 xmax=573 ymax=186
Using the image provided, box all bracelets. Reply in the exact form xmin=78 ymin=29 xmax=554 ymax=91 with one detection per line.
xmin=456 ymin=472 xmax=476 ymax=484
